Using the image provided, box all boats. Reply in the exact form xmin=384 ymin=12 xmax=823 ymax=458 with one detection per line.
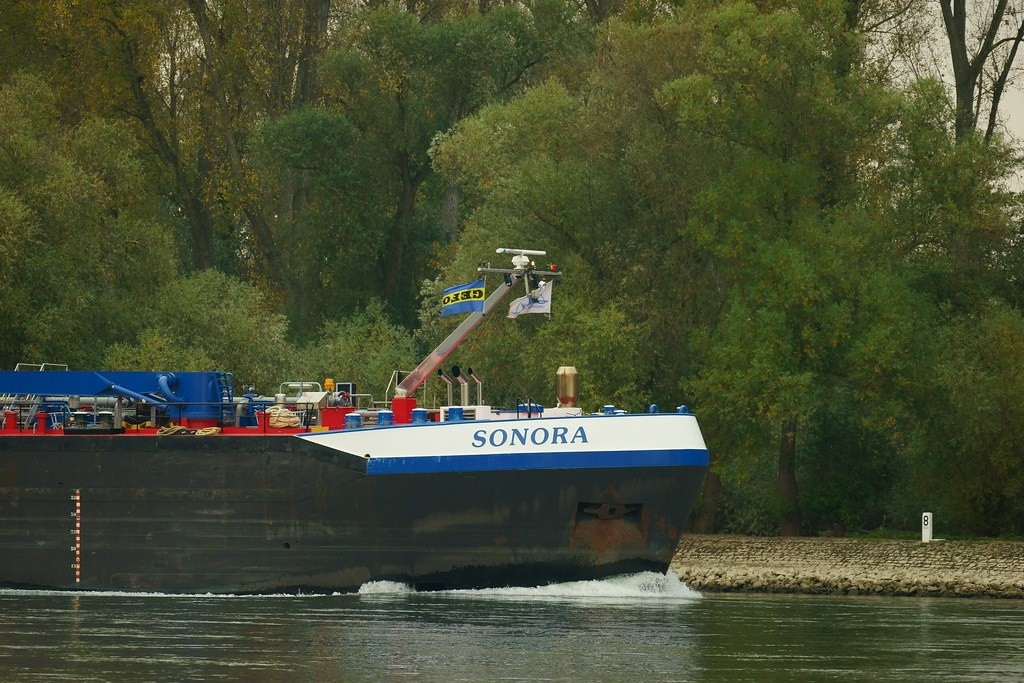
xmin=0 ymin=246 xmax=708 ymax=597
